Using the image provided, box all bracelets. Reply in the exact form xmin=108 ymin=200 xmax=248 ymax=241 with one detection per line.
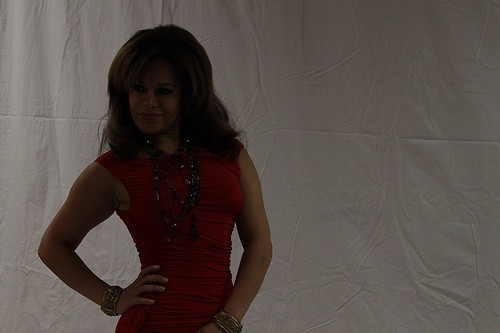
xmin=214 ymin=310 xmax=242 ymax=333
xmin=100 ymin=286 xmax=122 ymax=316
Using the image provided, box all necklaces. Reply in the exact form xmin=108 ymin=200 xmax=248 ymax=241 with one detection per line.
xmin=146 ymin=139 xmax=201 ymax=240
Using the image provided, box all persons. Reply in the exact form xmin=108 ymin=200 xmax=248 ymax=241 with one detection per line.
xmin=38 ymin=22 xmax=272 ymax=333
